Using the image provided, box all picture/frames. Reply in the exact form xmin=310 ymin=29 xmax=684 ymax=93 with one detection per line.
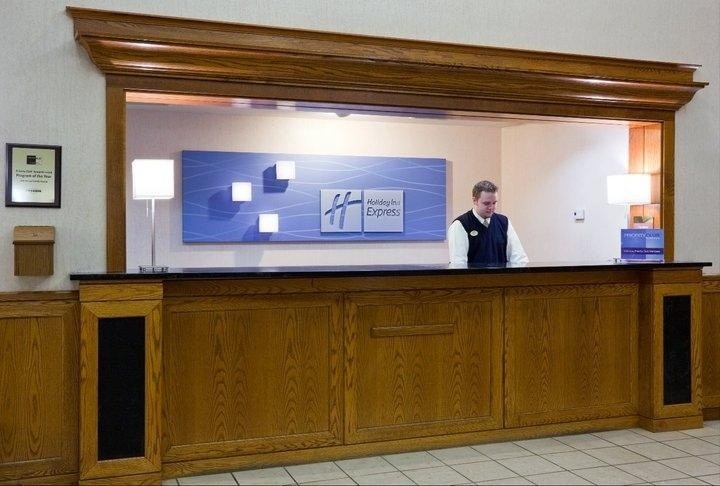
xmin=6 ymin=142 xmax=62 ymax=209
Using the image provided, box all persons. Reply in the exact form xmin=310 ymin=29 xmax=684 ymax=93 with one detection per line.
xmin=447 ymin=180 xmax=531 ymax=268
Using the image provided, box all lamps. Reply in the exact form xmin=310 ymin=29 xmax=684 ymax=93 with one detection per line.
xmin=130 ymin=156 xmax=176 ymax=271
xmin=607 ymin=170 xmax=652 ymax=229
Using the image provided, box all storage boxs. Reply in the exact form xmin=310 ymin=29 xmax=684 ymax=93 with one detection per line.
xmin=617 ymin=226 xmax=666 ymax=262
